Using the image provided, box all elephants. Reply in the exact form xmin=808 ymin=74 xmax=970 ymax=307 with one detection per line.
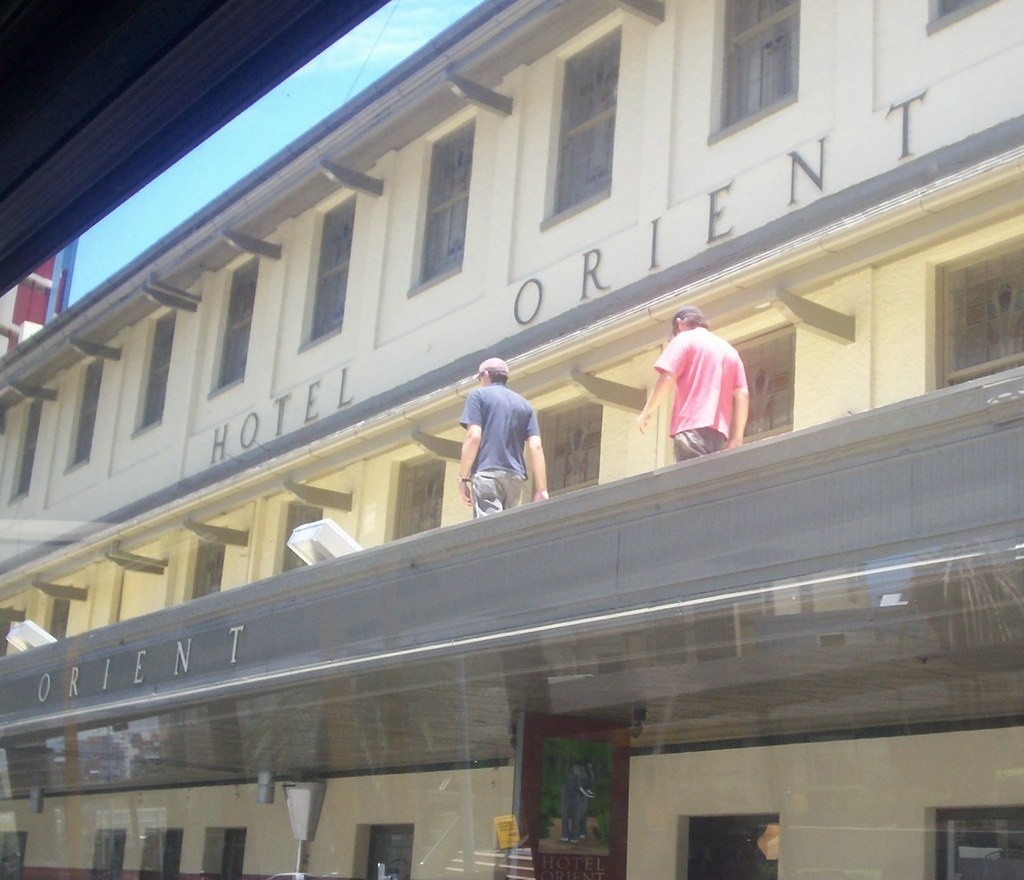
xmin=560 ymin=765 xmax=597 ymax=842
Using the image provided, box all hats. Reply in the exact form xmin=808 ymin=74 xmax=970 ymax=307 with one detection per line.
xmin=472 ymin=358 xmax=508 ymax=378
xmin=672 ymin=307 xmax=703 ymax=326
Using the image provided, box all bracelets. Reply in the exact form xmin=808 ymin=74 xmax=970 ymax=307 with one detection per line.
xmin=458 ymin=477 xmax=468 ymax=482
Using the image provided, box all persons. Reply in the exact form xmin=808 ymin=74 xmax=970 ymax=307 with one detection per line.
xmin=456 ymin=356 xmax=549 ymax=518
xmin=635 ymin=306 xmax=750 ymax=462
